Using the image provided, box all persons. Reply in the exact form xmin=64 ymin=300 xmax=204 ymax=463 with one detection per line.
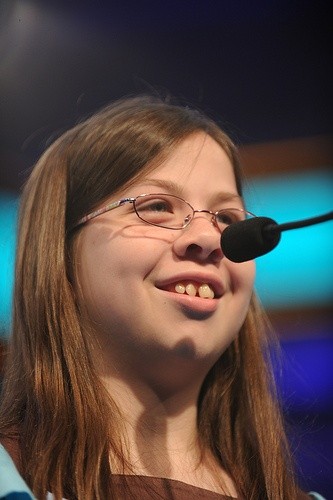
xmin=1 ymin=95 xmax=327 ymax=500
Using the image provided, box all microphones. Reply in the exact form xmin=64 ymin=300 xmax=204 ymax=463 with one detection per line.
xmin=220 ymin=211 xmax=333 ymax=263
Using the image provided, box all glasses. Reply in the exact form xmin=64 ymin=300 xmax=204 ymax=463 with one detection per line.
xmin=67 ymin=193 xmax=267 ymax=232
